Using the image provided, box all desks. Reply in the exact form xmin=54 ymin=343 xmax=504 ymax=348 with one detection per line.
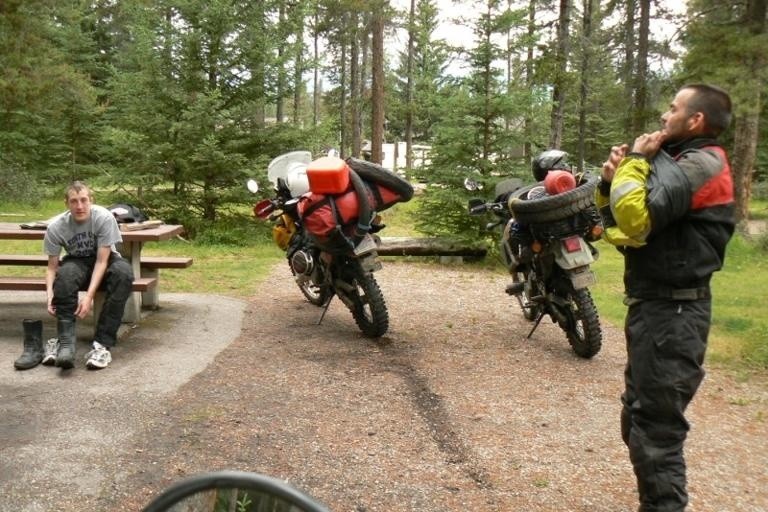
xmin=0 ymin=222 xmax=184 ymax=324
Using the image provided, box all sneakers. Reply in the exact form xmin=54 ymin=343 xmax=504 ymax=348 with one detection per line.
xmin=84 ymin=340 xmax=112 ymax=369
xmin=42 ymin=337 xmax=60 ymax=366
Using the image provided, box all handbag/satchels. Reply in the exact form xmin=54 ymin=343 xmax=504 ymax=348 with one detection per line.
xmin=297 ymin=171 xmax=401 ymax=243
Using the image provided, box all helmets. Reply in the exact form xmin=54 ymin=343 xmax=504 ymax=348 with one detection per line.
xmin=272 ymin=214 xmax=297 ymax=252
xmin=532 ymin=149 xmax=571 ymax=182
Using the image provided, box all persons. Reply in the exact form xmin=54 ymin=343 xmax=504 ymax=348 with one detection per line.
xmin=595 ymin=81 xmax=736 ymax=511
xmin=43 ymin=183 xmax=133 ymax=369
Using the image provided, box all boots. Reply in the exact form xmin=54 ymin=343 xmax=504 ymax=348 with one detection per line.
xmin=14 ymin=318 xmax=46 ymax=369
xmin=58 ymin=318 xmax=76 ymax=369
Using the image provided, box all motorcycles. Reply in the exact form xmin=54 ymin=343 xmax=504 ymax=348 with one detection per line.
xmin=464 ymin=150 xmax=604 ymax=357
xmin=246 ymin=149 xmax=412 ymax=339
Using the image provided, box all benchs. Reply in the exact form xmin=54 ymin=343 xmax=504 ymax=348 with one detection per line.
xmin=0 ymin=255 xmax=193 ymax=311
xmin=0 ymin=277 xmax=158 ymax=333
